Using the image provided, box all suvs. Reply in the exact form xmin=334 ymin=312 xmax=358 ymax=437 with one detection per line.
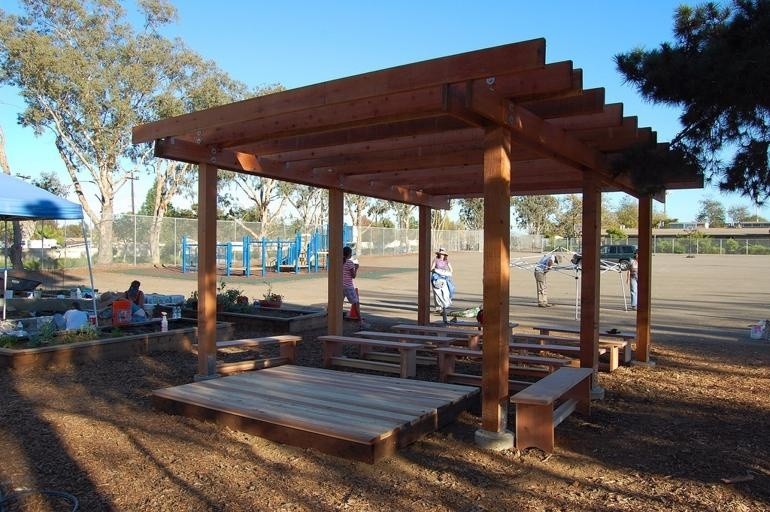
xmin=571 ymin=243 xmax=638 ymax=272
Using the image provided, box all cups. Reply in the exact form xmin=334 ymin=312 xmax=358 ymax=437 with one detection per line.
xmin=88 ymin=315 xmax=96 ymax=327
xmin=34 ymin=291 xmax=41 ymax=300
xmin=26 ymin=292 xmax=33 ymax=300
xmin=6 ymin=290 xmax=13 ymax=298
xmin=144 ymin=304 xmax=153 ymax=311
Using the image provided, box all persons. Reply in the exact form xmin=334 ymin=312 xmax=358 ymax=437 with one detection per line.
xmin=534 ymin=254 xmax=563 ymax=307
xmin=124 ymin=280 xmax=144 ymax=307
xmin=62 ymin=301 xmax=88 ymax=332
xmin=430 ymin=247 xmax=454 ymax=312
xmin=619 ymin=255 xmax=639 ymax=311
xmin=341 ymin=246 xmax=368 ymax=327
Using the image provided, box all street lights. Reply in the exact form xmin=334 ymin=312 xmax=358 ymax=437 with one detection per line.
xmin=61 ymin=178 xmax=97 ymax=287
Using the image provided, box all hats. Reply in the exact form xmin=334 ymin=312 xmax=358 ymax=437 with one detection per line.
xmin=556 ymin=255 xmax=562 ymax=264
xmin=100 ymin=292 xmax=121 ymax=308
xmin=435 ymin=248 xmax=448 ymax=256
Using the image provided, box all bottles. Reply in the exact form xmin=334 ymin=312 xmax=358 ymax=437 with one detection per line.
xmin=94 ymin=288 xmax=98 ymax=297
xmin=17 ymin=321 xmax=24 ymax=337
xmin=172 ymin=306 xmax=182 ymax=318
xmin=759 ymin=320 xmax=769 ymax=334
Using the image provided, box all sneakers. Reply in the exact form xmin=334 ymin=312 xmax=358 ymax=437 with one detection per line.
xmin=359 ymin=323 xmax=371 ymax=330
xmin=632 ymin=306 xmax=638 ymax=311
xmin=538 ymin=304 xmax=552 ymax=308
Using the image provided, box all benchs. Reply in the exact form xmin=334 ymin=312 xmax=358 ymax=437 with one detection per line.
xmin=190 ymin=334 xmax=304 ymax=373
xmin=509 ymin=365 xmax=595 ymax=454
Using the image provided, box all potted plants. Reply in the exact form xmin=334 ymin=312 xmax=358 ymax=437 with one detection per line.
xmin=258 ymin=280 xmax=283 ymax=309
